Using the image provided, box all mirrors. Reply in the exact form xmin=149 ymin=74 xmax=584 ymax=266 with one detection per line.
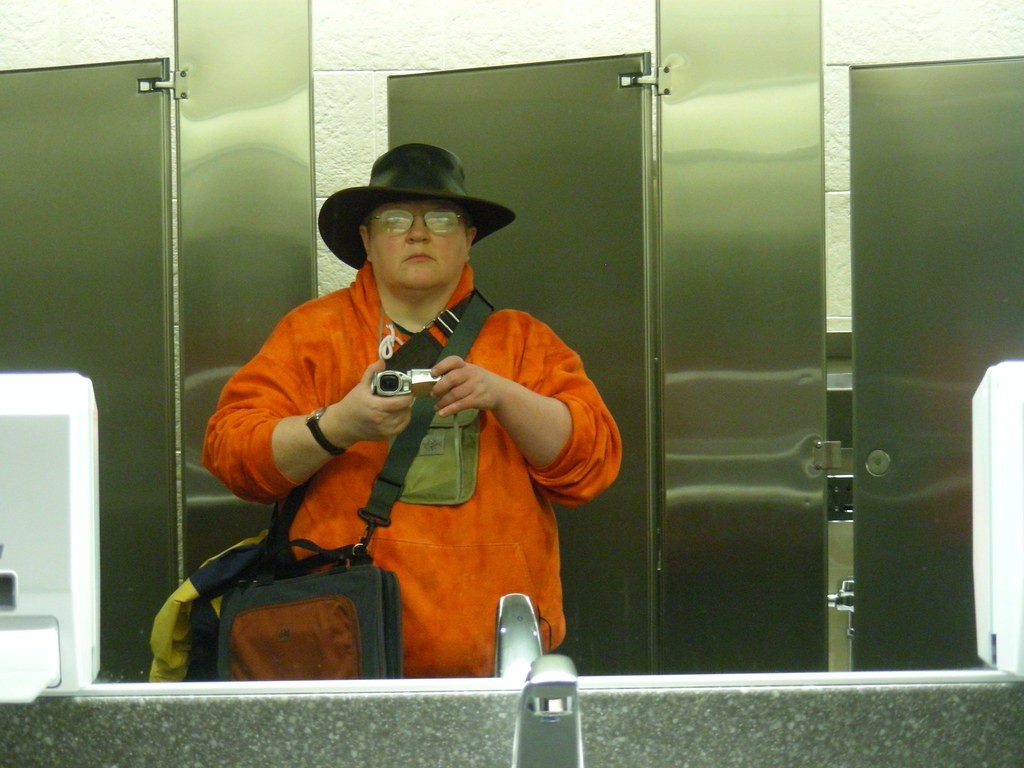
xmin=0 ymin=0 xmax=1024 ymax=683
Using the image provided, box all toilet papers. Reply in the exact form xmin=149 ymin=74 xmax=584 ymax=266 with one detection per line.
xmin=2 ymin=626 xmax=61 ymax=707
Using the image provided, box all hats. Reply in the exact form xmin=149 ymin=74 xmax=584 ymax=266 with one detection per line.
xmin=318 ymin=142 xmax=517 ymax=269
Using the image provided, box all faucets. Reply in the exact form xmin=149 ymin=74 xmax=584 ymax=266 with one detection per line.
xmin=512 ymin=653 xmax=586 ymax=768
xmin=491 ymin=591 xmax=543 ymax=677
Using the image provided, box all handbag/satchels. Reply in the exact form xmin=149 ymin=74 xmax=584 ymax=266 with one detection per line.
xmin=216 ymin=543 xmax=403 ymax=680
xmin=384 ymin=398 xmax=481 ymax=506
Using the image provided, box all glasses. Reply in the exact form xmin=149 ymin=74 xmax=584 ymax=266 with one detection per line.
xmin=364 ymin=208 xmax=471 ymax=235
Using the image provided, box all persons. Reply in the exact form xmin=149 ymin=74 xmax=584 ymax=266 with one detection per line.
xmin=202 ymin=143 xmax=623 ymax=678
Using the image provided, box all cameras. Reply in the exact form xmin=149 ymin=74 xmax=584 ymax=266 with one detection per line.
xmin=372 ymin=368 xmax=444 ymax=398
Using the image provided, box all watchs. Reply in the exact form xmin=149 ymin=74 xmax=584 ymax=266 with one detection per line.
xmin=305 ymin=406 xmax=347 ymax=455
